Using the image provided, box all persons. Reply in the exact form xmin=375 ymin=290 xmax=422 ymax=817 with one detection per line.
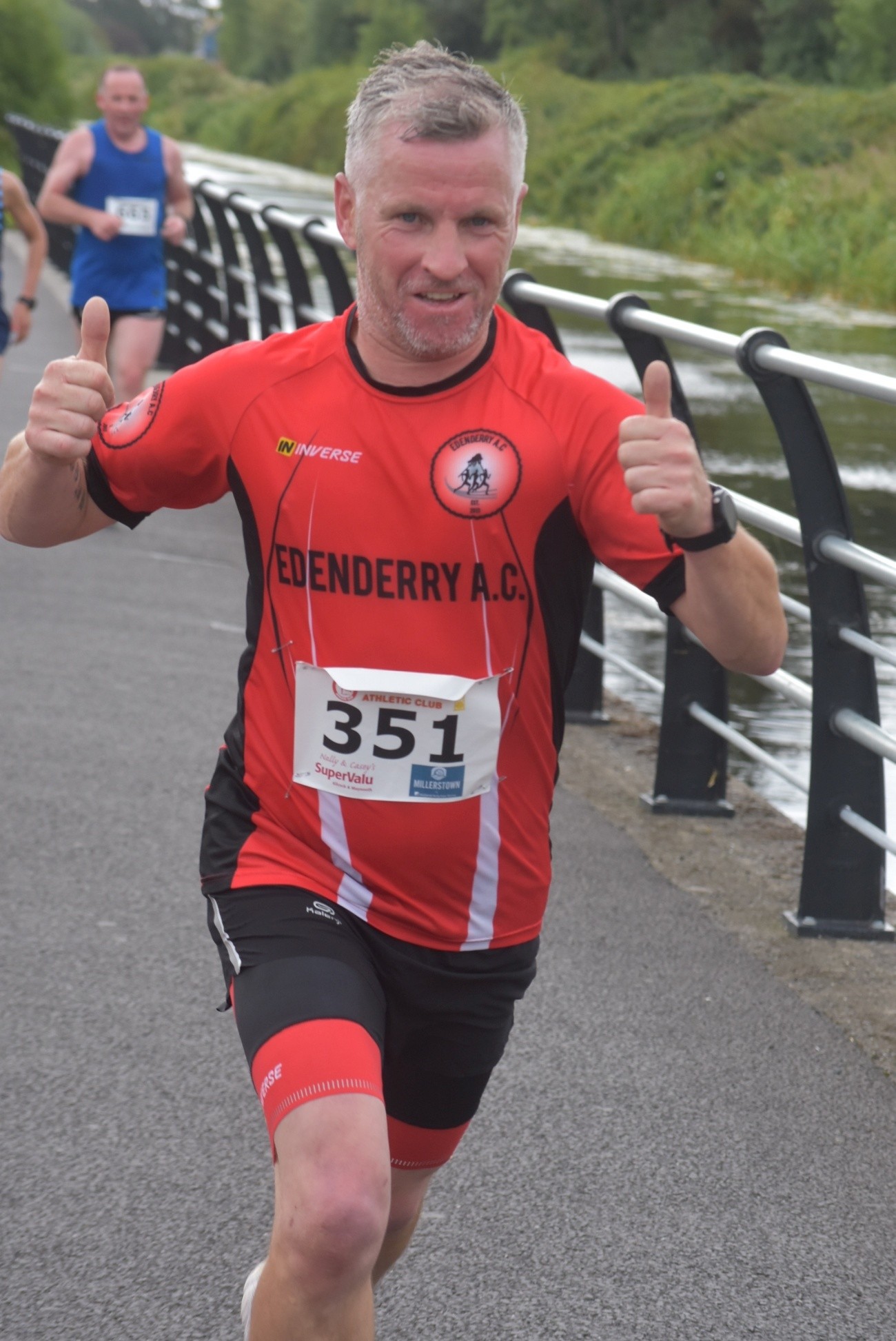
xmin=0 ymin=167 xmax=47 ymax=363
xmin=38 ymin=63 xmax=193 ymax=411
xmin=0 ymin=40 xmax=789 ymax=1341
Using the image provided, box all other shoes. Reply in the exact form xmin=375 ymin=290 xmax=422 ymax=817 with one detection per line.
xmin=239 ymin=1258 xmax=265 ymax=1341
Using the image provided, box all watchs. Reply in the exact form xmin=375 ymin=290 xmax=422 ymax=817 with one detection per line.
xmin=17 ymin=295 xmax=41 ymax=310
xmin=659 ymin=482 xmax=738 ymax=553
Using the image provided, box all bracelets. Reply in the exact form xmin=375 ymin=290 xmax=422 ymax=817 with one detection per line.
xmin=172 ymin=208 xmax=194 ymax=236
